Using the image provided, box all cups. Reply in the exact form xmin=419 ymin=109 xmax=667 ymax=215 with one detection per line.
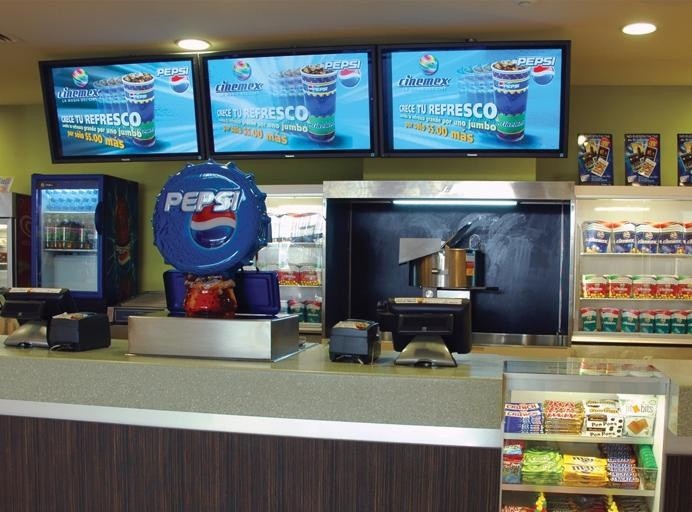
xmin=301 ymin=65 xmax=338 ymax=143
xmin=489 ymin=64 xmax=530 ymax=142
xmin=121 ymin=73 xmax=155 ymax=146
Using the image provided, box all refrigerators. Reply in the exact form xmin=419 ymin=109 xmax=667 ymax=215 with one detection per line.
xmin=30 ymin=173 xmax=138 ymax=310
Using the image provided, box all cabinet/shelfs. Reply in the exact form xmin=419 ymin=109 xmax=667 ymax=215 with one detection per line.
xmin=499 ymin=359 xmax=670 ymax=512
xmin=570 ymin=193 xmax=691 ymax=345
xmin=242 ymin=184 xmax=325 ymax=334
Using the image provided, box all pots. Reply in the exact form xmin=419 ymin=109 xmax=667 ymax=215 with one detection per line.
xmin=414 ymin=245 xmax=469 ymax=290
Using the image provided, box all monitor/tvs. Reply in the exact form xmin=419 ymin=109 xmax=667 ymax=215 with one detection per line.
xmin=377 ymin=38 xmax=571 ymax=159
xmin=38 ymin=53 xmax=205 ymax=164
xmin=198 ymin=45 xmax=378 ymax=161
xmin=0 ymin=286 xmax=79 ymax=349
xmin=387 ymin=297 xmax=474 ymax=367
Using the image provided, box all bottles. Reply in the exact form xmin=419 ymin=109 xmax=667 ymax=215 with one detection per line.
xmin=44 ymin=217 xmax=94 ymax=248
